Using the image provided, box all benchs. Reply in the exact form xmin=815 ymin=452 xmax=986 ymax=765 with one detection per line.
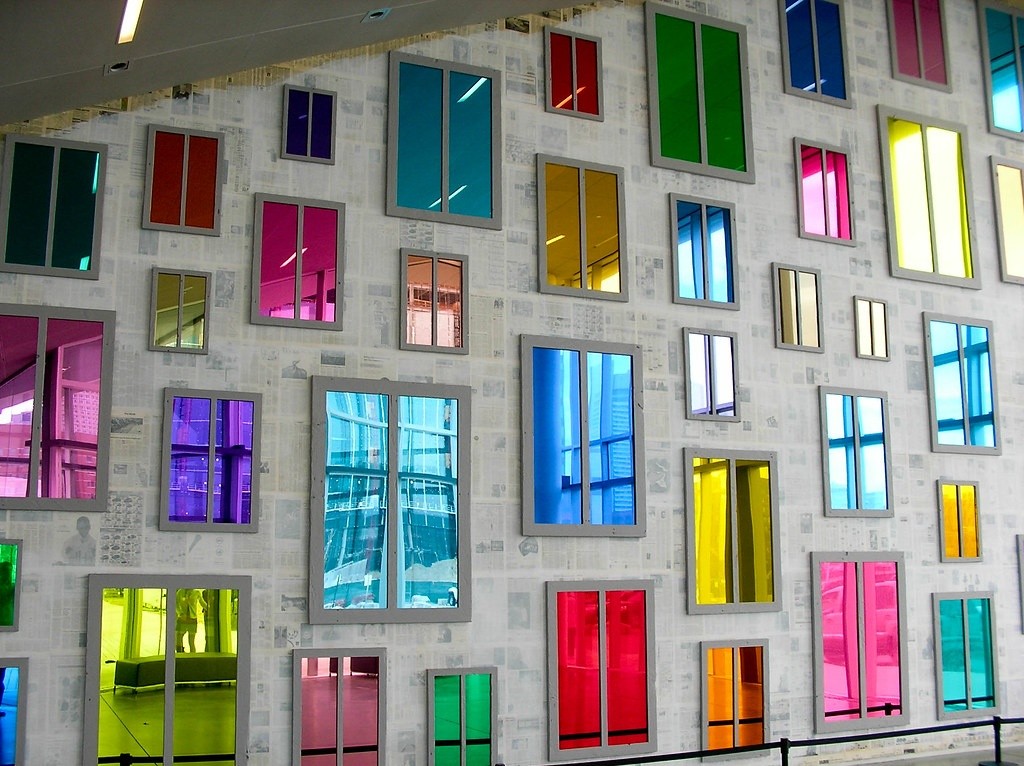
xmin=113 ymin=651 xmax=236 ymax=696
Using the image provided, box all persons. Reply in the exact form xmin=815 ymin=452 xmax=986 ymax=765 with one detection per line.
xmin=175 ymin=588 xmax=210 ymax=654
xmin=61 ymin=516 xmax=95 ymax=567
